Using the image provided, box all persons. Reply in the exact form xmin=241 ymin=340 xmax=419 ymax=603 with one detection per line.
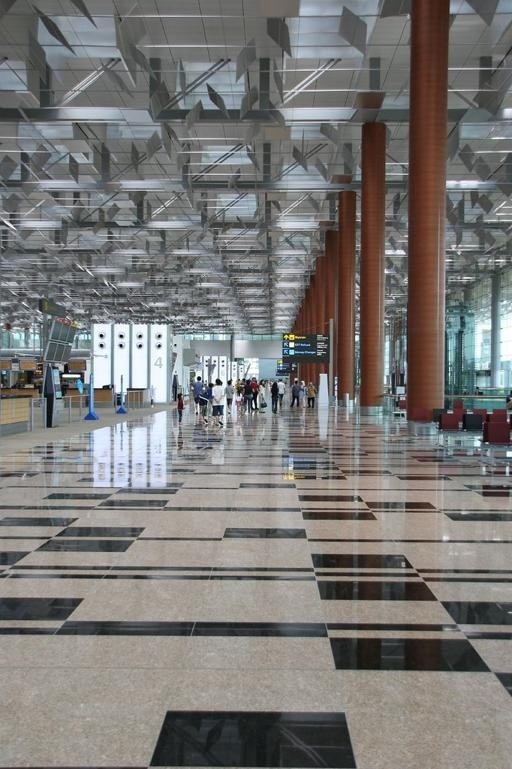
xmin=504 ymin=391 xmax=512 ymax=411
xmin=176 ymin=392 xmax=184 ymax=424
xmin=307 ymin=381 xmax=317 ymax=409
xmin=176 ymin=410 xmax=318 ymax=454
xmin=193 ymin=373 xmax=307 ymax=427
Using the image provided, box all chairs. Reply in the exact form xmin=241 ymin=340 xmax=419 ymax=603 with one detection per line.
xmin=432 ymin=399 xmax=512 ymax=446
xmin=392 ymin=399 xmax=407 ymax=420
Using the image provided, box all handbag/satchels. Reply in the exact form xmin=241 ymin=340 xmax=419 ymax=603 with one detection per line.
xmin=261 ymin=403 xmax=267 ymax=408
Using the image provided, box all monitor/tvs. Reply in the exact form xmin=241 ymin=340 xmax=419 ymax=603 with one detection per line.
xmin=42 ymin=341 xmax=57 ymax=363
xmin=50 ymin=319 xmax=63 ymax=342
xmin=66 ymin=325 xmax=76 ymax=343
xmin=58 ymin=324 xmax=69 ymax=343
xmin=62 ymin=345 xmax=72 ymax=362
xmin=54 ymin=343 xmax=66 ymax=363
